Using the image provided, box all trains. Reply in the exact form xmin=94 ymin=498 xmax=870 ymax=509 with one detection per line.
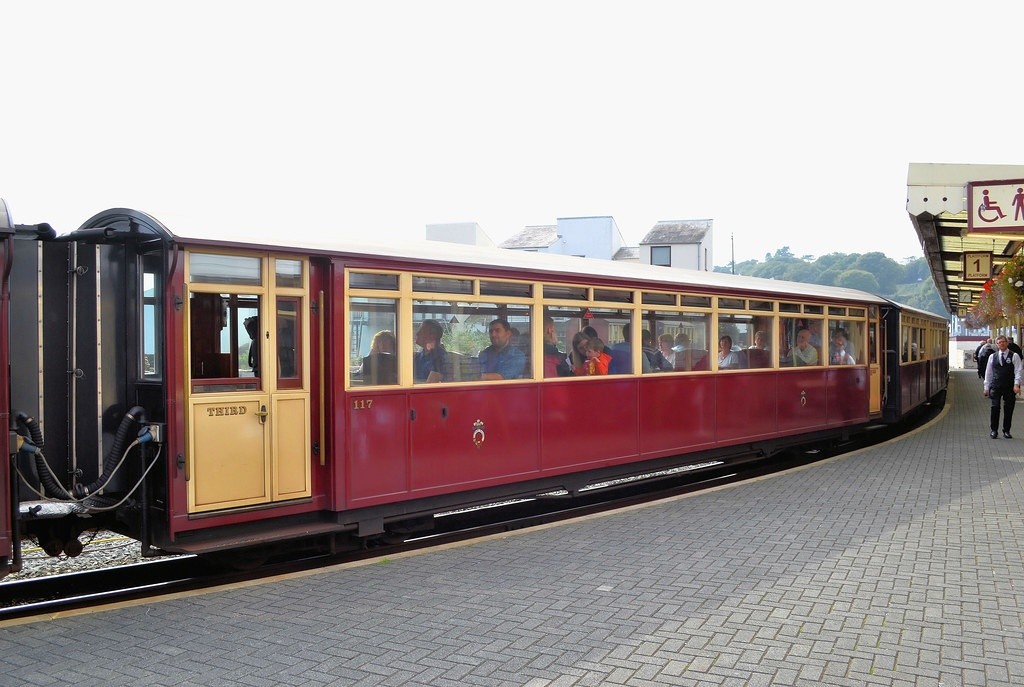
xmin=0 ymin=207 xmax=951 ymax=580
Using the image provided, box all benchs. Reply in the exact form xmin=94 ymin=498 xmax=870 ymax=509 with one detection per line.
xmin=682 ymin=349 xmax=708 ymax=371
xmin=743 ymin=348 xmax=771 ymax=368
xmin=614 ymin=349 xmax=630 ymax=374
xmin=446 ymin=351 xmax=462 ymax=382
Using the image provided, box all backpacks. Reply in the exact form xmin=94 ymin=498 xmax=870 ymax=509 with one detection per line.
xmin=985 ymin=344 xmax=996 ymax=363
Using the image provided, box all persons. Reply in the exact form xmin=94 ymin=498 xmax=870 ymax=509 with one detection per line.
xmin=566 ymin=325 xmax=613 ymax=375
xmin=413 ymin=319 xmax=452 ymax=383
xmin=829 ymin=328 xmax=856 ymax=365
xmin=983 ymin=335 xmax=1022 ymax=439
xmin=359 ymin=329 xmax=396 ymax=372
xmin=478 ymin=318 xmax=526 ymax=380
xmin=787 ymin=320 xmax=821 ymax=366
xmin=719 ymin=335 xmax=739 ymax=370
xmin=975 ymin=337 xmax=1022 ymax=381
xmin=623 ymin=323 xmax=691 ymax=373
xmin=543 ymin=316 xmax=575 ymax=378
xmin=902 ymin=343 xmax=917 ymax=362
xmin=748 ymin=331 xmax=771 ymax=350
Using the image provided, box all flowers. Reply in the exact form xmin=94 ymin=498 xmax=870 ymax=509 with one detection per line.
xmin=965 ymin=252 xmax=1024 ymax=329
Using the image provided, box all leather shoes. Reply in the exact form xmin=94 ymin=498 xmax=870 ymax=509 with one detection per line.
xmin=1002 ymin=431 xmax=1012 ymax=438
xmin=990 ymin=430 xmax=998 ymax=439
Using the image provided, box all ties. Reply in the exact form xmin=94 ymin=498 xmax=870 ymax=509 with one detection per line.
xmin=1001 ymin=353 xmax=1006 ymax=365
